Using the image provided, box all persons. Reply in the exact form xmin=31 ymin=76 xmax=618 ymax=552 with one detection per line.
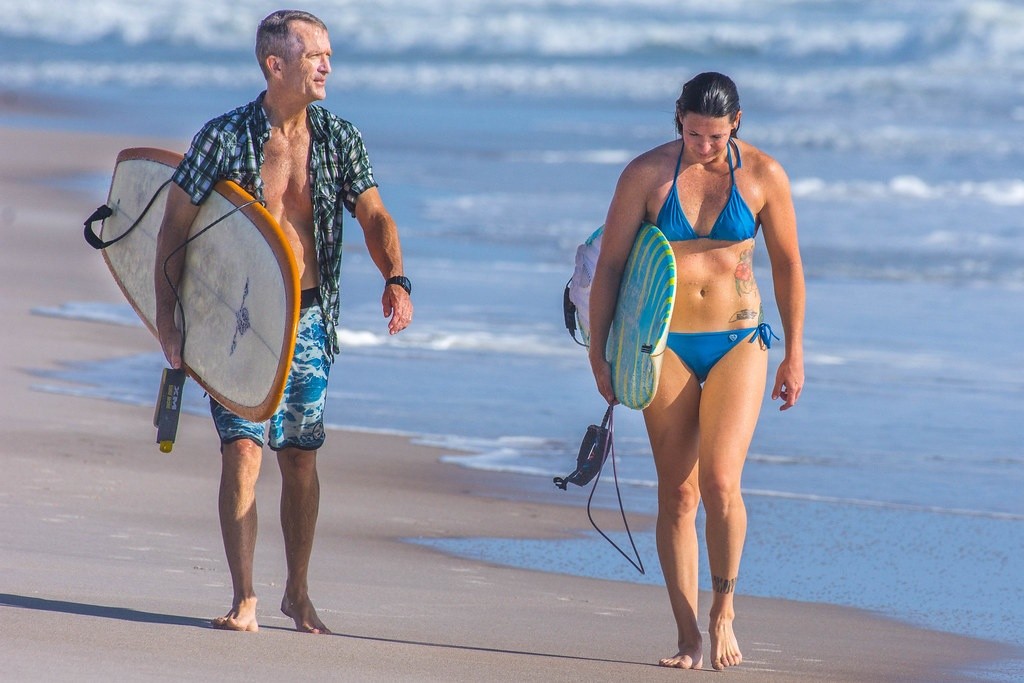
xmin=587 ymin=70 xmax=807 ymax=672
xmin=154 ymin=9 xmax=414 ymax=634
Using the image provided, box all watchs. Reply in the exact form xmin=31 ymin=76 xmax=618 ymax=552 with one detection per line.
xmin=385 ymin=276 xmax=411 ymax=294
